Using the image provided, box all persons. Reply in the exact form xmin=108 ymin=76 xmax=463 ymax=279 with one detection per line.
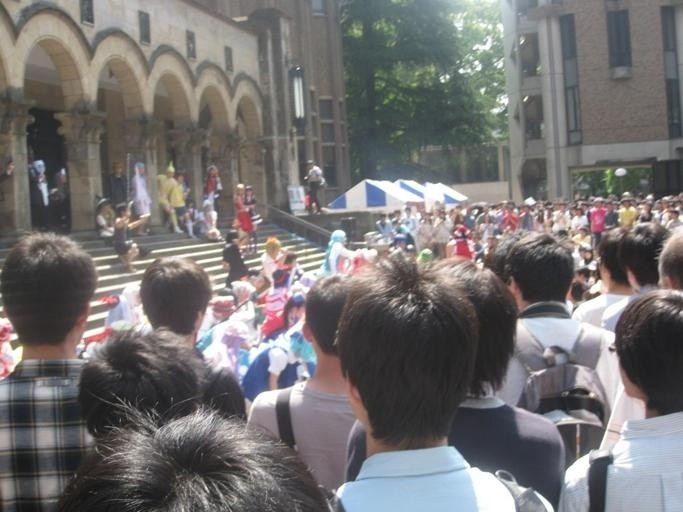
xmin=128 ymin=162 xmax=151 ymax=236
xmin=113 ymin=200 xmax=149 ymax=272
xmin=56 ymin=394 xmax=333 ymax=511
xmin=245 ymin=275 xmax=363 ymax=495
xmin=78 ymin=325 xmax=248 ymax=435
xmin=571 ymin=227 xmax=634 ymax=324
xmin=502 ymin=236 xmax=622 ymax=435
xmin=375 ymin=191 xmax=490 ymax=266
xmin=108 ymin=162 xmax=128 ymax=203
xmin=158 ymin=161 xmax=224 ymax=242
xmin=657 ymin=231 xmax=683 ymax=293
xmin=324 ymin=230 xmax=374 ymax=266
xmin=233 ymin=183 xmax=256 ymax=246
xmin=0 ymin=161 xmax=15 ymax=238
xmin=489 ymin=191 xmax=681 ymax=238
xmin=559 ymin=288 xmax=683 ymax=512
xmin=244 ymin=186 xmax=263 ymax=246
xmin=199 ymin=229 xmax=315 ymax=402
xmin=94 ymin=199 xmax=153 ymax=258
xmin=30 ymin=161 xmax=70 ymax=232
xmin=604 ymin=223 xmax=671 ymax=332
xmin=303 ymin=160 xmax=327 ymax=214
xmin=1 ymin=233 xmax=99 ymax=511
xmin=139 ymin=256 xmax=246 ymax=417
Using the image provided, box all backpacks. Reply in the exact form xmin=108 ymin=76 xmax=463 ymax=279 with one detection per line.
xmin=513 ymin=318 xmax=612 ymax=460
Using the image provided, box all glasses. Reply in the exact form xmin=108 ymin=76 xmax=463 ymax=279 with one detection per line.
xmin=609 ymin=341 xmax=617 ymax=357
